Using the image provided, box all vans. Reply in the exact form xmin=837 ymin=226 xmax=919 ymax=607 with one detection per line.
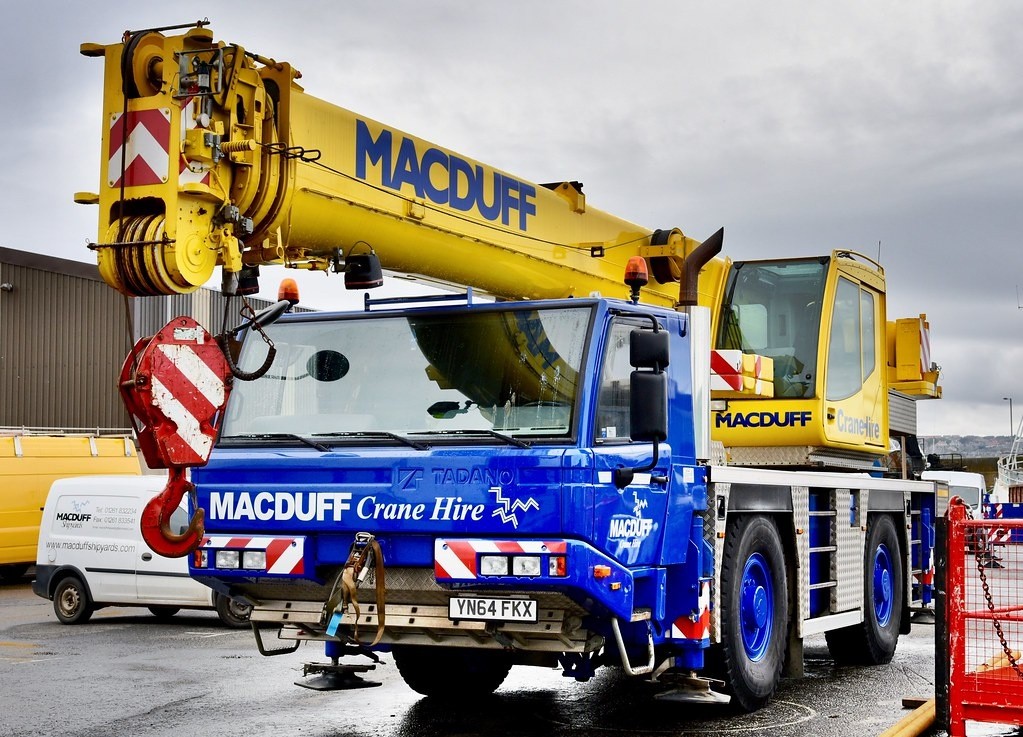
xmin=30 ymin=474 xmax=259 ymax=628
xmin=0 ymin=433 xmax=145 ymax=585
xmin=918 ymin=470 xmax=990 ymax=553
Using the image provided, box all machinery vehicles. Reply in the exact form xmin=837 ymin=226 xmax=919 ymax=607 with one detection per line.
xmin=76 ymin=16 xmax=949 ymax=717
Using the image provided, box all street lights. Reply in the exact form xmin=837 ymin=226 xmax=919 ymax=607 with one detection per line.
xmin=1002 ymin=397 xmax=1017 ymax=442
